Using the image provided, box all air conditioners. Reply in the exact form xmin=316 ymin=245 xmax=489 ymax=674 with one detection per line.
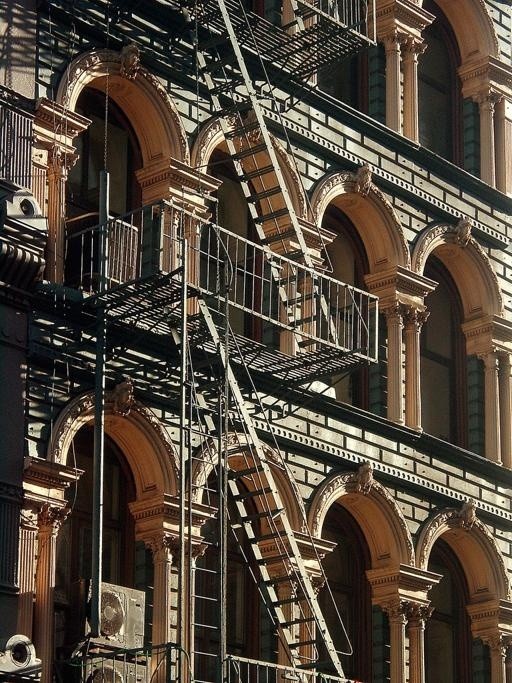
xmin=75 ymin=579 xmax=145 ymax=652
xmin=78 ymin=658 xmax=147 ymax=683
